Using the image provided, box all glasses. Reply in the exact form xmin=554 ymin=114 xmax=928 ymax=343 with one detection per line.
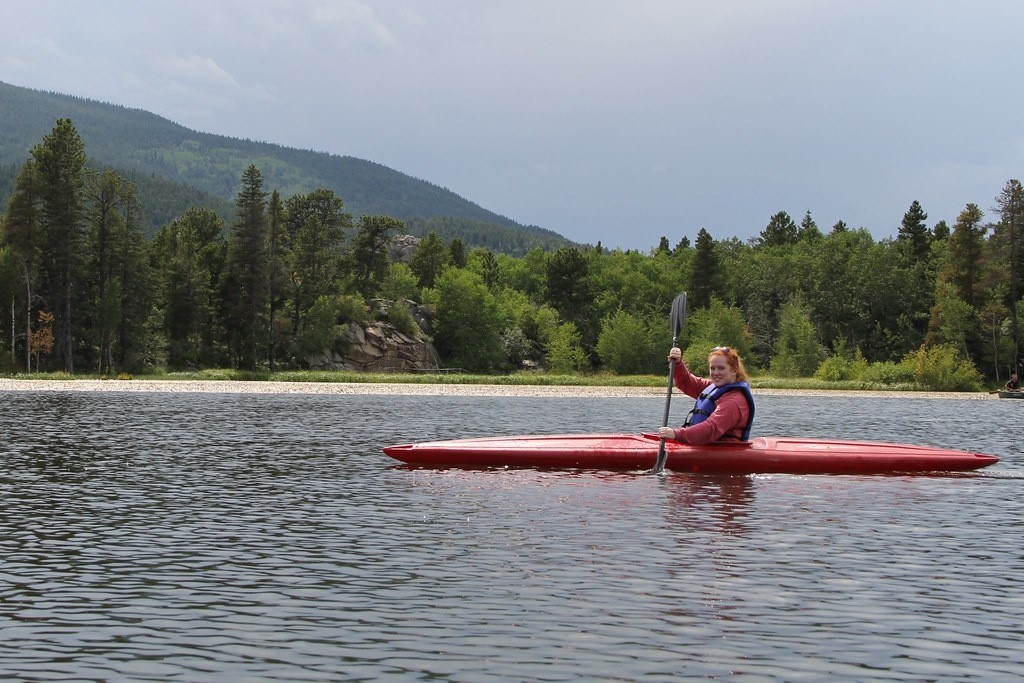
xmin=710 ymin=346 xmax=734 ymax=356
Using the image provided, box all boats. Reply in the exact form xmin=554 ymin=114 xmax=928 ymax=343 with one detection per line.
xmin=381 ymin=430 xmax=1001 ymax=475
xmin=997 ymin=388 xmax=1024 ymax=399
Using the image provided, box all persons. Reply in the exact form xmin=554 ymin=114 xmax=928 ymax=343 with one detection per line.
xmin=659 ymin=346 xmax=755 ymax=443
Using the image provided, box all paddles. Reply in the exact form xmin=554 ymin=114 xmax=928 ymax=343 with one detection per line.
xmin=655 ymin=292 xmax=687 ymax=474
xmin=989 ymin=389 xmax=1024 ymax=394
